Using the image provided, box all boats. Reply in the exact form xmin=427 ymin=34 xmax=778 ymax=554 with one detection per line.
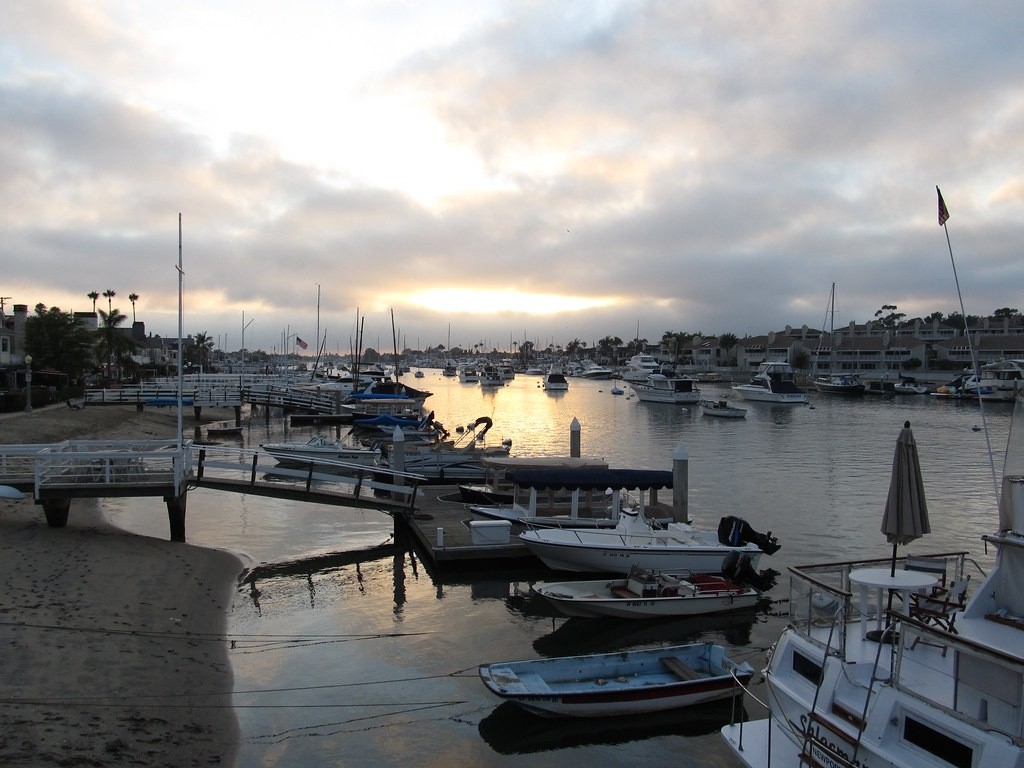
xmin=519 ymin=494 xmax=783 ymax=576
xmin=141 ymin=285 xmax=1024 ymax=528
xmin=474 ymin=639 xmax=754 ymax=729
xmin=527 ymin=567 xmax=762 ymax=626
xmin=721 ymin=181 xmax=1024 ymax=768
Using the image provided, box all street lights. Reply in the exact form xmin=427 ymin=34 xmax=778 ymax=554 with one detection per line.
xmin=23 ymin=354 xmax=34 ymax=413
